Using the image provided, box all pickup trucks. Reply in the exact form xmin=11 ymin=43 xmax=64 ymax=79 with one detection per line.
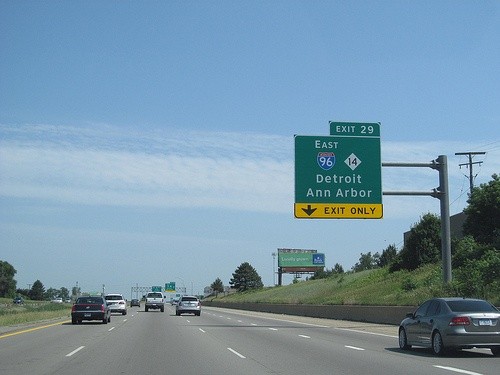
xmin=142 ymin=292 xmax=166 ymax=312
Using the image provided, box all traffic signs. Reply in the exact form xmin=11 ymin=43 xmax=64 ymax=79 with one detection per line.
xmin=164 ymin=283 xmax=175 ymax=292
xmin=152 ymin=286 xmax=162 ymax=292
xmin=293 ymin=134 xmax=383 ymax=219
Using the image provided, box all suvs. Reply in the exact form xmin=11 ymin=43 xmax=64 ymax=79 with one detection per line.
xmin=102 ymin=294 xmax=127 ymax=315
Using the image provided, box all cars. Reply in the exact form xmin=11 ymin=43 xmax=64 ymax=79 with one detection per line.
xmin=131 ymin=299 xmax=141 ymax=307
xmin=176 ymin=295 xmax=202 ymax=316
xmin=51 ymin=297 xmax=71 ymax=303
xmin=398 ymin=297 xmax=500 ymax=357
xmin=71 ymin=296 xmax=112 ymax=324
xmin=13 ymin=297 xmax=23 ymax=304
